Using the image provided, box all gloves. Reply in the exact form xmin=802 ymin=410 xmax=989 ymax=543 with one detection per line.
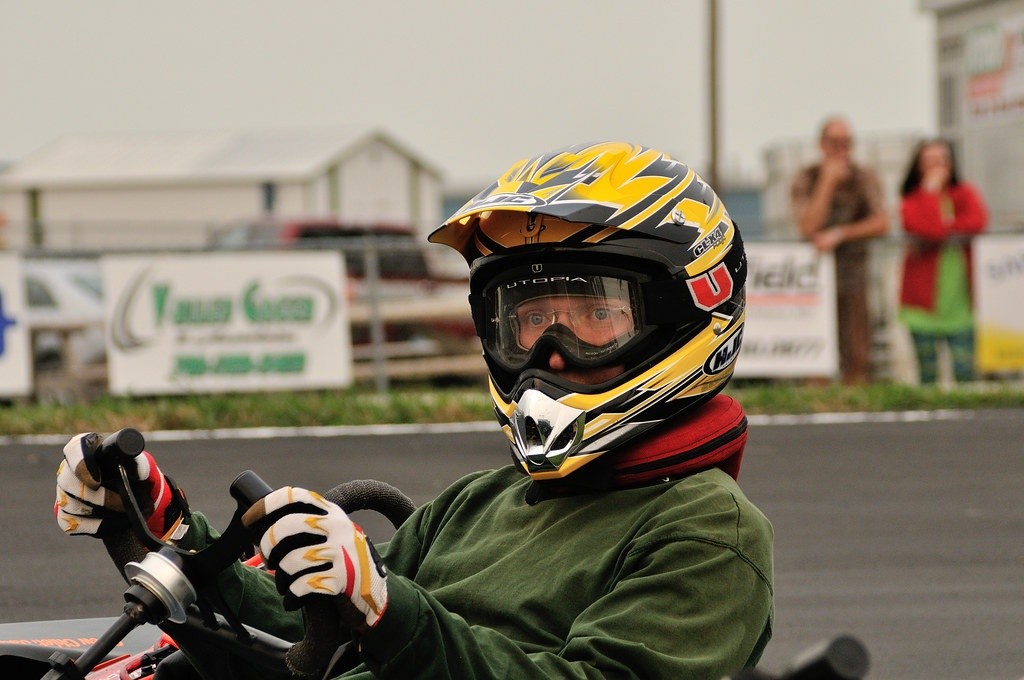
xmin=242 ymin=484 xmax=389 ymax=627
xmin=53 ymin=429 xmax=196 ymax=581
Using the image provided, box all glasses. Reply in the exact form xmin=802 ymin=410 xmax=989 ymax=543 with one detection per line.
xmin=505 ymin=300 xmax=632 ymax=336
xmin=824 ymin=137 xmax=851 ymax=147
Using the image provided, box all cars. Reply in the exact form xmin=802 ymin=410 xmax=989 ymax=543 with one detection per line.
xmin=21 ymin=260 xmax=109 ymax=403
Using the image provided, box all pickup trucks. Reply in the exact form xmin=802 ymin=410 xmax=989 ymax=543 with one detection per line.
xmin=204 ymin=219 xmax=478 ymax=355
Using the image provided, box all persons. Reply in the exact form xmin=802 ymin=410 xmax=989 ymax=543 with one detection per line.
xmin=792 ymin=119 xmax=886 ymax=390
xmin=899 ymin=137 xmax=987 ymax=388
xmin=54 ymin=139 xmax=774 ymax=680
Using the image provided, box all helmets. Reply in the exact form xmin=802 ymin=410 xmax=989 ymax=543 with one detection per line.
xmin=429 ymin=137 xmax=746 ymax=479
